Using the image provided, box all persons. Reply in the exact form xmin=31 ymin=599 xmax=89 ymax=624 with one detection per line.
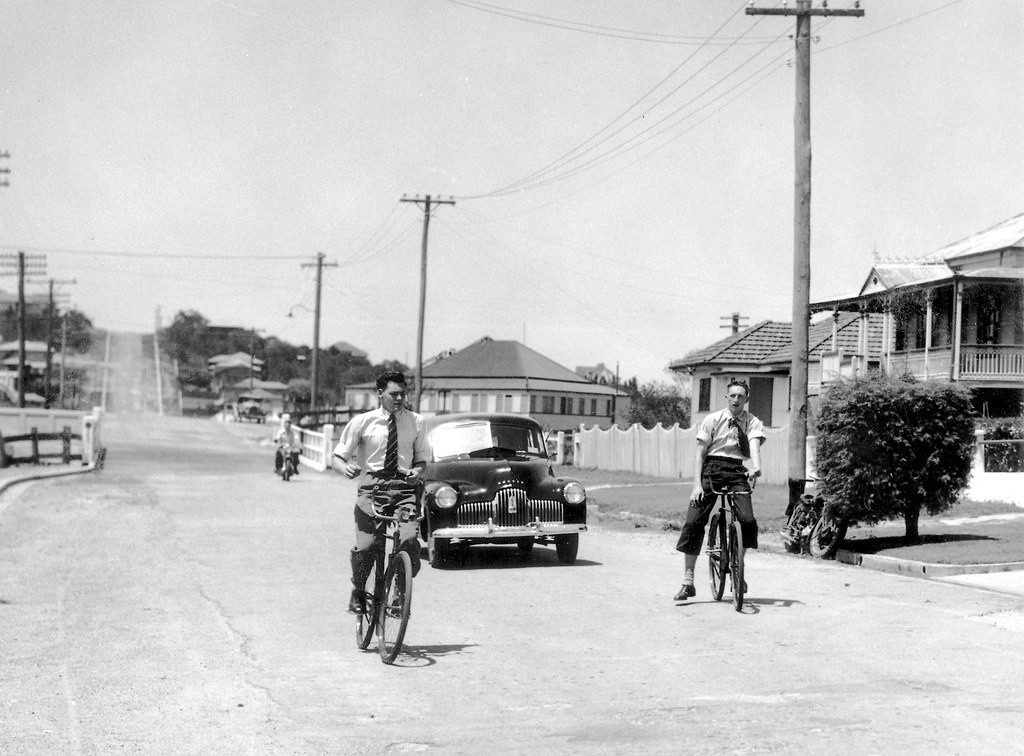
xmin=330 ymin=371 xmax=428 ymax=615
xmin=274 ymin=421 xmax=301 ymax=475
xmin=674 ymin=380 xmax=767 ymax=600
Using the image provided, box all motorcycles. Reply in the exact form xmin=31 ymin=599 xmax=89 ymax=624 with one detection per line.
xmin=778 ymin=479 xmax=848 ymax=559
xmin=274 ymin=440 xmax=303 ymax=481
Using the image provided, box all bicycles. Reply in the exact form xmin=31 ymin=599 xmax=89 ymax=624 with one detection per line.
xmin=346 ymin=486 xmax=425 ymax=665
xmin=700 ymin=471 xmax=758 ymax=612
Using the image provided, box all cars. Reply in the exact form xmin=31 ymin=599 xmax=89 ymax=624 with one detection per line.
xmin=419 ymin=412 xmax=588 ymax=569
xmin=236 ymin=393 xmax=267 ymax=424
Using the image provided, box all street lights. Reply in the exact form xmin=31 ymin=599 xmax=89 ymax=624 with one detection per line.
xmin=287 ymin=303 xmax=320 ymax=411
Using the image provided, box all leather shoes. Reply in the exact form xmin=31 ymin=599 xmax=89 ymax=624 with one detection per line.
xmin=349 ymin=591 xmax=364 ymax=615
xmin=673 ymin=586 xmax=696 ymax=602
xmin=392 ymin=599 xmax=409 ymax=616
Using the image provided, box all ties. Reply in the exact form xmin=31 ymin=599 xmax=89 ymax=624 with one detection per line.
xmin=383 ymin=412 xmax=397 ymax=482
xmin=729 ymin=418 xmax=751 ymax=460
